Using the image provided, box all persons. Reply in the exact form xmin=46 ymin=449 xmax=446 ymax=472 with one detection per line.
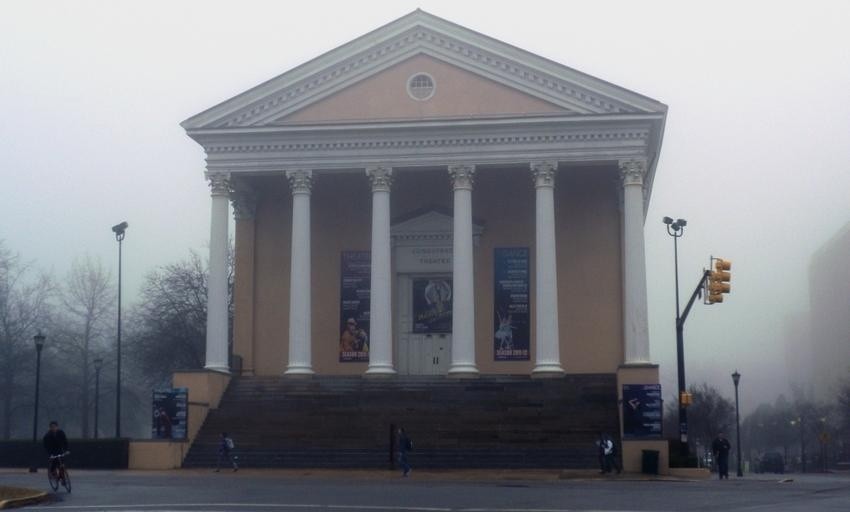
xmin=211 ymin=432 xmax=239 ymax=473
xmin=711 ymin=432 xmax=732 ymax=481
xmin=43 ymin=421 xmax=72 ymax=486
xmin=601 ymin=431 xmax=622 ymax=475
xmin=340 ymin=317 xmax=368 ymax=355
xmin=501 ymin=313 xmax=518 ymax=350
xmin=494 ymin=309 xmax=510 ymax=356
xmin=397 ymin=427 xmax=413 ymax=479
xmin=596 ymin=431 xmax=609 ymax=475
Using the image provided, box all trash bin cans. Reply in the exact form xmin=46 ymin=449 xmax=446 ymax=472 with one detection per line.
xmin=642 ymin=449 xmax=659 ymax=475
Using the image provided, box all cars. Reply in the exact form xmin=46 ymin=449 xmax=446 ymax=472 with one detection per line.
xmin=702 ymin=451 xmax=712 ymax=467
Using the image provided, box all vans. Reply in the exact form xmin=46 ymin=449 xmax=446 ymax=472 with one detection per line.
xmin=753 ymin=450 xmax=785 ymax=474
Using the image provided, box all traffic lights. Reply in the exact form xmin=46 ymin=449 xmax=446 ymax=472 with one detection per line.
xmin=710 ymin=254 xmax=734 ymax=293
xmin=705 ymin=269 xmax=723 ymax=307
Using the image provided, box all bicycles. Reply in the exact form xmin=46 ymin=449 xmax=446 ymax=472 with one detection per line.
xmin=47 ymin=451 xmax=72 ymax=492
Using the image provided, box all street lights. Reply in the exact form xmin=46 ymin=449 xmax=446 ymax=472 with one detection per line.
xmin=818 ymin=414 xmax=827 ymax=472
xmin=730 ymin=369 xmax=742 ymax=477
xmin=93 ymin=354 xmax=104 ymax=438
xmin=662 ymin=216 xmax=689 ymax=431
xmin=111 ymin=220 xmax=129 ymax=436
xmin=789 ymin=414 xmax=806 ymax=474
xmin=28 ymin=326 xmax=49 ymax=473
